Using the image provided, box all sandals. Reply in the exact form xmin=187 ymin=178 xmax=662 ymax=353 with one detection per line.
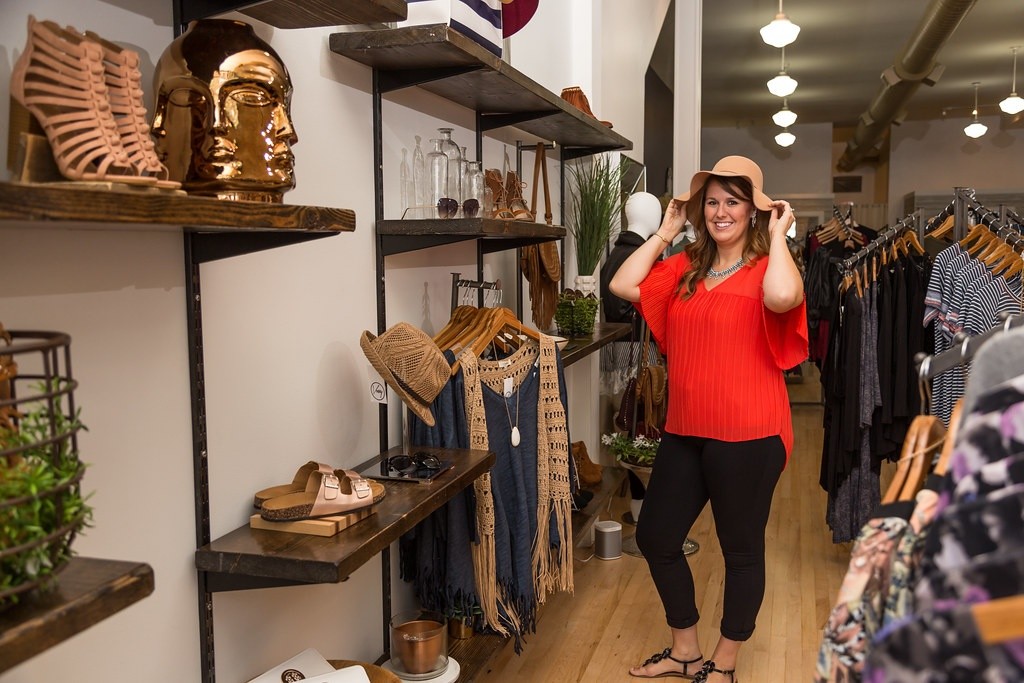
xmin=691 ymin=660 xmax=738 ymax=683
xmin=628 ymin=647 xmax=705 ymax=679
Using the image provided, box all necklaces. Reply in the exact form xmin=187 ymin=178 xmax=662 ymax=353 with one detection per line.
xmin=706 ymin=257 xmax=744 ymax=279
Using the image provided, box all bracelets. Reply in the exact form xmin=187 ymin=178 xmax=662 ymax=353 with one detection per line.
xmin=654 ymin=233 xmax=670 ymax=245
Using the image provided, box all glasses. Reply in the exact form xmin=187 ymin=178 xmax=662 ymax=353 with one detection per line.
xmin=385 ymin=450 xmax=441 ymax=479
xmin=401 ymin=197 xmax=482 ymax=220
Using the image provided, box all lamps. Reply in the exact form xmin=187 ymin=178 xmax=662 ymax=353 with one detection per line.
xmin=759 ymin=0 xmax=801 ymax=49
xmin=771 ymin=95 xmax=798 ymax=128
xmin=964 ymin=82 xmax=988 ymax=139
xmin=774 ymin=125 xmax=796 ymax=148
xmin=998 ymin=45 xmax=1024 ymax=115
xmin=766 ymin=47 xmax=798 ymax=98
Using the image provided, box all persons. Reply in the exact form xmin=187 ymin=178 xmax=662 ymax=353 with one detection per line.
xmin=611 ymin=155 xmax=809 ymax=683
xmin=150 ymin=18 xmax=299 ymax=201
xmin=599 ymin=191 xmax=662 ymax=410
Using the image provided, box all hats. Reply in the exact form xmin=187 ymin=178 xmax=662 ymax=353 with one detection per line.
xmin=672 ymin=155 xmax=779 ymax=233
xmin=358 ymin=321 xmax=453 ymax=428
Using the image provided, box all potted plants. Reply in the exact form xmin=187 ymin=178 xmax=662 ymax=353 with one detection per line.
xmin=561 ymin=151 xmax=635 ymax=298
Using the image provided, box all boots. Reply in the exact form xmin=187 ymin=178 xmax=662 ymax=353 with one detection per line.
xmin=505 ymin=169 xmax=536 ymax=223
xmin=567 ymin=440 xmax=605 ymax=495
xmin=482 ymin=166 xmax=517 ymax=222
xmin=560 ymin=85 xmax=614 ymax=130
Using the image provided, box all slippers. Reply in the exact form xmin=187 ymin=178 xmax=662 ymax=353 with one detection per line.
xmin=253 ymin=469 xmax=387 ymax=522
xmin=253 ymin=461 xmax=360 ymax=510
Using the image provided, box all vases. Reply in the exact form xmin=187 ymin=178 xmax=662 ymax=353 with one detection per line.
xmin=618 ymin=460 xmax=653 ymax=491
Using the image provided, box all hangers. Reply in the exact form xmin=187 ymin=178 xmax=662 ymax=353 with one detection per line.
xmin=431 ymin=280 xmax=541 ymax=378
xmin=924 ymin=188 xmax=1024 ymax=282
xmin=969 ymin=593 xmax=1024 ymax=647
xmin=816 ymin=206 xmax=924 ymax=299
xmin=879 ymin=313 xmax=1014 ymax=505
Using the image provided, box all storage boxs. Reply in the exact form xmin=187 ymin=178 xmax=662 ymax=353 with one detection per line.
xmin=396 ymin=0 xmax=502 ymax=58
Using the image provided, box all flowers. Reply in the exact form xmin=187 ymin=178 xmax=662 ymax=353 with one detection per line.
xmin=600 ymin=431 xmax=662 ymax=467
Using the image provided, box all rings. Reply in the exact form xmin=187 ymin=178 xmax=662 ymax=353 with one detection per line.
xmin=791 ymin=208 xmax=794 ymax=211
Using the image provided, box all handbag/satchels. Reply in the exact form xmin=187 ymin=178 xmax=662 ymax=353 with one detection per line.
xmin=519 ymin=141 xmax=563 ymax=331
xmin=613 ymin=305 xmax=670 ymax=448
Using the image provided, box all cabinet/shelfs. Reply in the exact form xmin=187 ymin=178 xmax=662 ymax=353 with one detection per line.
xmin=1 ymin=0 xmax=633 ymax=683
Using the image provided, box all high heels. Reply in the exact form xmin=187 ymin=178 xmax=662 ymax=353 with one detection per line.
xmin=5 ymin=13 xmax=158 ymax=188
xmin=63 ymin=22 xmax=183 ymax=190
